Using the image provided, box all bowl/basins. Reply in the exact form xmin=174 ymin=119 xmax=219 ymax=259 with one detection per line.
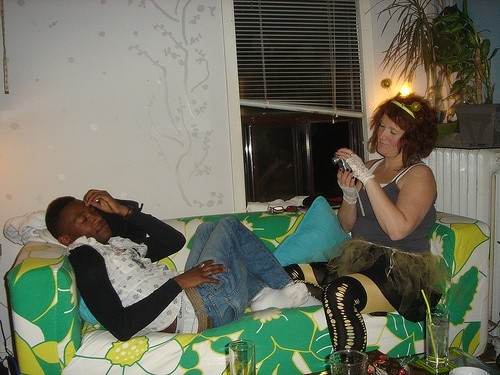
xmin=449 ymin=366 xmax=491 ymax=375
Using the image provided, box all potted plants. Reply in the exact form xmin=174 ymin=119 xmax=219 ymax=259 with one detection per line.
xmin=364 ymin=0 xmax=500 ymax=148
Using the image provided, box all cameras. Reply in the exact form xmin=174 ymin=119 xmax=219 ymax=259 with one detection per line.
xmin=331 ymin=156 xmax=352 ymax=173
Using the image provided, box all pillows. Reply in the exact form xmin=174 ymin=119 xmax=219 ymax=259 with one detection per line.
xmin=272 ymin=196 xmax=352 ymax=267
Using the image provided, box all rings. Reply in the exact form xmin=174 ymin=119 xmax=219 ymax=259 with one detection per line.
xmin=207 ymin=270 xmax=211 ymax=274
xmin=200 ymin=263 xmax=205 ymax=267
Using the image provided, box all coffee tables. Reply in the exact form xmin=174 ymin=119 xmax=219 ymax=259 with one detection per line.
xmin=305 ymin=347 xmax=500 ymax=375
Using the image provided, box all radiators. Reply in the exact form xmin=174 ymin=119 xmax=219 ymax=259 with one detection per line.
xmin=421 ymin=148 xmax=500 ymax=357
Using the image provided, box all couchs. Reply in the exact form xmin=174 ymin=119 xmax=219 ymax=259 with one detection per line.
xmin=5 ymin=207 xmax=491 ymax=375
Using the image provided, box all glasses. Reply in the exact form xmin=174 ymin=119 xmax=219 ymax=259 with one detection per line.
xmin=270 ymin=206 xmax=297 ymax=214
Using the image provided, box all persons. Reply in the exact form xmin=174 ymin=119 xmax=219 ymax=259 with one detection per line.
xmin=45 ymin=189 xmax=309 ymax=342
xmin=281 ymin=94 xmax=444 ymax=367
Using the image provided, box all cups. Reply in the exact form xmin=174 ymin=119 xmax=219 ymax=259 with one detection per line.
xmin=329 ymin=350 xmax=368 ymax=375
xmin=426 ymin=308 xmax=449 ymax=368
xmin=229 ymin=339 xmax=256 ymax=375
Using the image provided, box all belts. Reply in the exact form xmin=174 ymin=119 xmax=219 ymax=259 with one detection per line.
xmin=185 ymin=287 xmax=208 ymax=333
